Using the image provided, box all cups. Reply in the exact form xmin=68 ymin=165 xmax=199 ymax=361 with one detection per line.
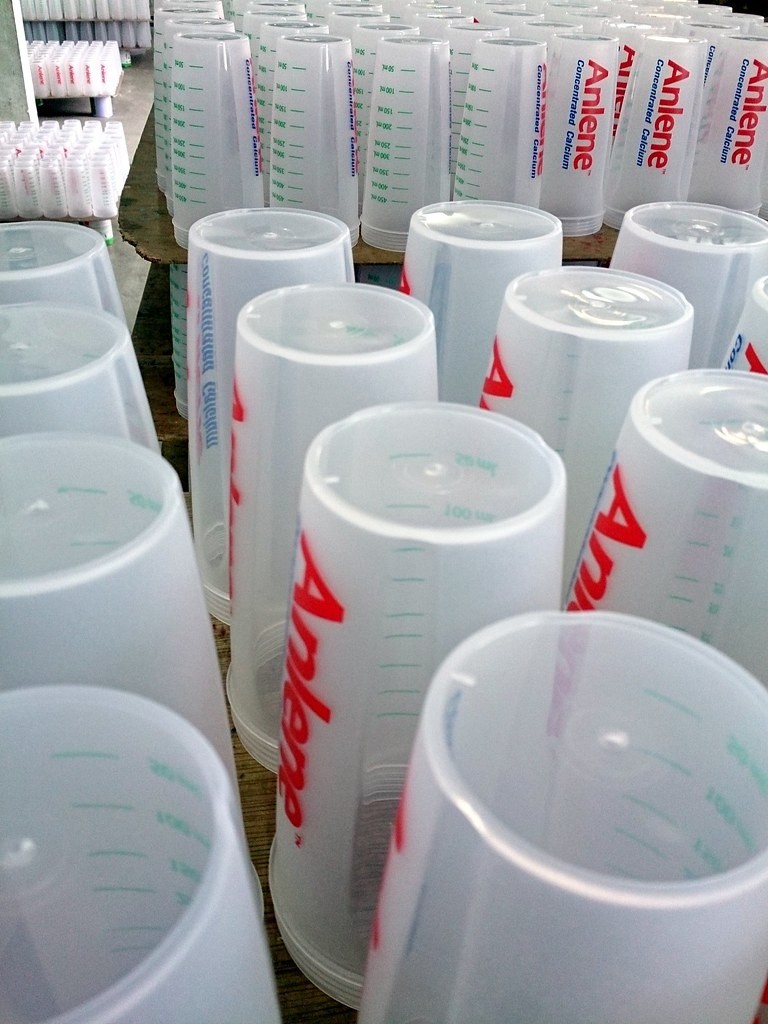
xmin=1 ymin=0 xmax=768 ymax=1024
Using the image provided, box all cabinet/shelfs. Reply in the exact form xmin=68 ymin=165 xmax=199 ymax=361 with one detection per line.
xmin=114 ymin=102 xmax=620 ymax=443
xmin=23 ymin=19 xmax=153 ymax=68
